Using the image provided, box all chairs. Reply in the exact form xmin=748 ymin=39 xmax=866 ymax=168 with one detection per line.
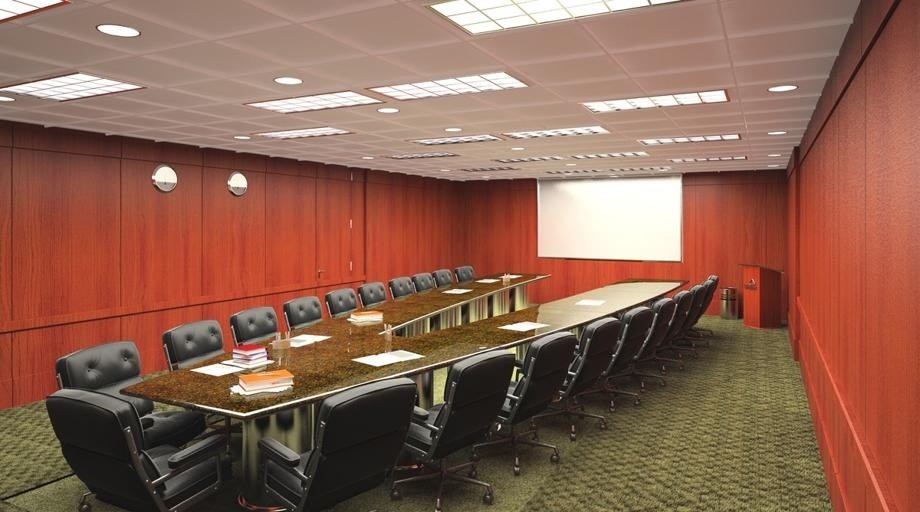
xmin=42 ymin=260 xmax=719 ymax=510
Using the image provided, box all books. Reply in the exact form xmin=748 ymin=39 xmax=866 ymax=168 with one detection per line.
xmin=239 ymin=369 xmax=294 ymax=392
xmin=351 ymin=310 xmax=383 ymax=320
xmin=233 ymin=344 xmax=267 ymax=365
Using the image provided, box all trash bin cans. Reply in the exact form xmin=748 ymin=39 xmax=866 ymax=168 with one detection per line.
xmin=720 ymin=287 xmax=738 ymax=320
